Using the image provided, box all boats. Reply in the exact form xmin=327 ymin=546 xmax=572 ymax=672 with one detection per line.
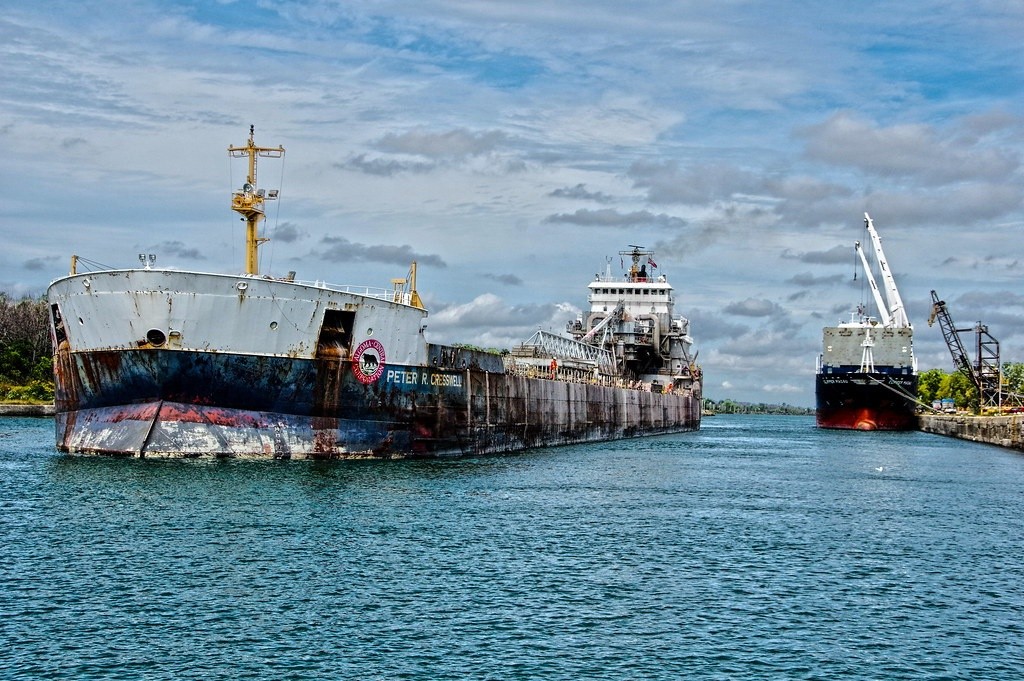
xmin=43 ymin=126 xmax=702 ymax=463
xmin=815 ymin=213 xmax=919 ymax=432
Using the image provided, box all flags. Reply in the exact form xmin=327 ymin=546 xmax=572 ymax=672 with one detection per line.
xmin=621 ymin=257 xmax=623 ymax=269
xmin=648 ymin=257 xmax=657 ymax=268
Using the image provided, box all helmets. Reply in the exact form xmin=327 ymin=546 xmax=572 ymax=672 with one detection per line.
xmin=553 ymin=357 xmax=556 ymax=360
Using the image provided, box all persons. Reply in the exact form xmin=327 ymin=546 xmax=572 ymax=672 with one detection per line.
xmin=668 ymin=382 xmax=673 ymax=394
xmin=551 ymin=358 xmax=557 ymax=380
